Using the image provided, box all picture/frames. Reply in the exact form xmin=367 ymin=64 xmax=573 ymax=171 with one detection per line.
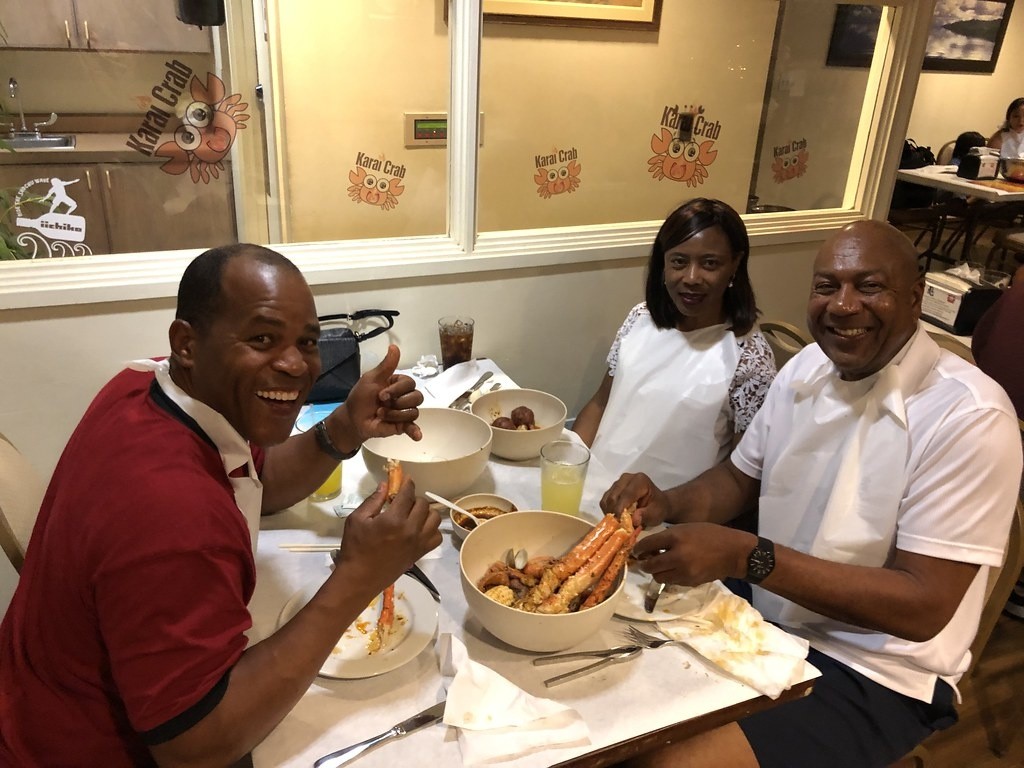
xmin=443 ymin=0 xmax=663 ymax=32
xmin=826 ymin=1 xmax=1016 ymax=73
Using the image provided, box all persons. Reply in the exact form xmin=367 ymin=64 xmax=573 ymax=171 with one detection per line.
xmin=0 ymin=242 xmax=442 ymax=768
xmin=597 ymin=220 xmax=1023 ymax=768
xmin=935 ymin=97 xmax=1024 ymax=221
xmin=571 ymin=197 xmax=778 ymax=527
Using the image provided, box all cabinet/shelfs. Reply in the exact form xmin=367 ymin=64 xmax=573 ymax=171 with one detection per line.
xmin=0 ymin=164 xmax=238 ymax=260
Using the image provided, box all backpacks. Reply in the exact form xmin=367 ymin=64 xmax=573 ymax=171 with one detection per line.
xmin=891 ymin=139 xmax=936 ymax=208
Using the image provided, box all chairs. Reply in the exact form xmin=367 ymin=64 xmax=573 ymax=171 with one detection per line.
xmin=912 ymin=131 xmax=1023 ymax=279
xmin=888 ymin=499 xmax=1024 ymax=768
xmin=758 ymin=321 xmax=815 ymax=372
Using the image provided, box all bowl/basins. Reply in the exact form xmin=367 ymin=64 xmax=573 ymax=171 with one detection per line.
xmin=471 ymin=388 xmax=567 ymax=461
xmin=449 ymin=495 xmax=519 ymax=540
xmin=1001 ymin=157 xmax=1024 ymax=179
xmin=460 ymin=510 xmax=628 ymax=652
xmin=362 ymin=407 xmax=493 ymax=503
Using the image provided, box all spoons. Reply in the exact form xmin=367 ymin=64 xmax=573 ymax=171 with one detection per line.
xmin=425 ymin=491 xmax=487 ymax=525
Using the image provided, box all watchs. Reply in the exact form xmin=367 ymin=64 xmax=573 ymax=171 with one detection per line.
xmin=741 ymin=536 xmax=777 ymax=586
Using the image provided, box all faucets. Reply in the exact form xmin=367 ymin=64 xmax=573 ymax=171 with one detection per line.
xmin=9 ymin=77 xmax=28 ymax=132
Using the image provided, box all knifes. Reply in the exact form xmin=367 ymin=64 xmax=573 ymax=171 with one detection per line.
xmin=314 ymin=701 xmax=444 ymax=768
xmin=449 ymin=371 xmax=493 ymax=409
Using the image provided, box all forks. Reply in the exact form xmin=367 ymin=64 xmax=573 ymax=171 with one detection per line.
xmin=625 ymin=624 xmax=673 ymax=649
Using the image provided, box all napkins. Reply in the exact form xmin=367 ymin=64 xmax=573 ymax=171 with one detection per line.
xmin=656 ymin=577 xmax=810 ymax=701
xmin=437 ymin=658 xmax=590 ymax=766
xmin=424 ymin=358 xmax=497 ymax=410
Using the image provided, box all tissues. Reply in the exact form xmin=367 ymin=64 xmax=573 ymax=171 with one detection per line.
xmin=918 ymin=261 xmax=1004 ymax=337
xmin=956 ymin=146 xmax=1001 ymax=180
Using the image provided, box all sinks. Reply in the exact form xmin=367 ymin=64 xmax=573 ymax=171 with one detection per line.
xmin=0 ymin=133 xmax=77 ymax=149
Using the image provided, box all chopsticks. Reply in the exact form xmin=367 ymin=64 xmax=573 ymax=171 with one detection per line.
xmin=278 ymin=543 xmax=341 ymax=552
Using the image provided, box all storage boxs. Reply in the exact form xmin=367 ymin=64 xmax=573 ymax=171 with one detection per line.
xmin=920 ymin=270 xmax=1003 ymax=337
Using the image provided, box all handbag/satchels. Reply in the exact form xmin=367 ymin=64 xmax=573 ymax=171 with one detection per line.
xmin=310 ymin=308 xmax=402 ymax=403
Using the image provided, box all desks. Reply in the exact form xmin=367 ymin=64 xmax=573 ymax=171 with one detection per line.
xmin=897 ymin=162 xmax=1024 ymax=281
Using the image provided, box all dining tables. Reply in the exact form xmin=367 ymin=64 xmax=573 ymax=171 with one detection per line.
xmin=232 ymin=356 xmax=821 ymax=767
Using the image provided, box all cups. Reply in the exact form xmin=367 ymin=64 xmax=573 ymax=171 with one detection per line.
xmin=955 ymin=261 xmax=1011 ymax=288
xmin=295 ymin=411 xmax=342 ymax=500
xmin=540 ymin=440 xmax=591 ymax=517
xmin=438 ymin=316 xmax=475 ymax=371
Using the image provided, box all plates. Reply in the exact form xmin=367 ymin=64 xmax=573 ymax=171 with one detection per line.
xmin=277 ymin=574 xmax=438 ymax=679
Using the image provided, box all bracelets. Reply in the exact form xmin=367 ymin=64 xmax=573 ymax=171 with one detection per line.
xmin=314 ymin=421 xmax=361 ymax=460
